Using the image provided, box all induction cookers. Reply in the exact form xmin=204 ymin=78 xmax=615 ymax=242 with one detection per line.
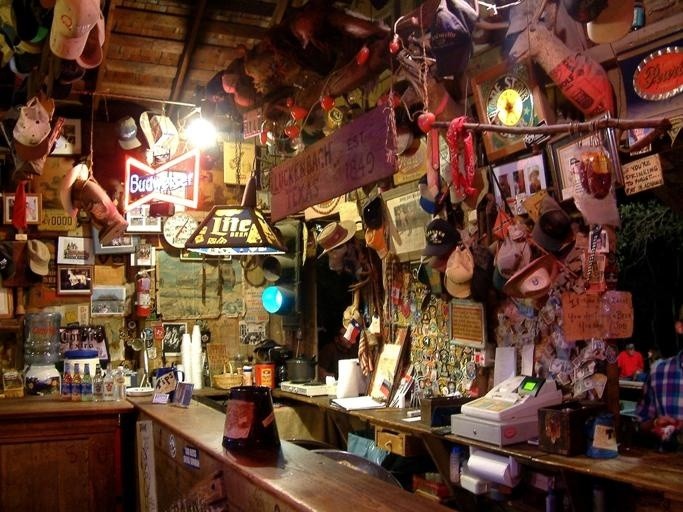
xmin=281 ymin=380 xmax=332 ymax=396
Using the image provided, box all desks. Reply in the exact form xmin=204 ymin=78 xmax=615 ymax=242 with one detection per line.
xmin=361 ymin=408 xmax=683 ymax=512
xmin=126 ymin=387 xmax=465 ymax=512
xmin=275 ymin=386 xmax=422 ymax=478
xmin=0 ymin=388 xmax=134 ymax=511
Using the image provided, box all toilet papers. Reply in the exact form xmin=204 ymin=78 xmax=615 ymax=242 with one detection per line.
xmin=466 ymin=449 xmax=521 ymax=489
xmin=337 ymin=358 xmax=360 ymax=400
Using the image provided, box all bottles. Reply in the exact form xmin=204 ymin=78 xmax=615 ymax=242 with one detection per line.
xmin=81 ymin=364 xmax=93 ymax=401
xmin=92 ymin=364 xmax=104 ymax=401
xmin=243 ymin=366 xmax=253 ymax=387
xmin=50 ymin=377 xmax=60 ymax=400
xmin=115 ymin=366 xmax=127 ymax=402
xmin=104 ymin=363 xmax=115 ymax=401
xmin=61 ymin=363 xmax=73 ymax=401
xmin=448 ymin=446 xmax=461 ymax=483
xmin=71 ymin=363 xmax=82 ymax=401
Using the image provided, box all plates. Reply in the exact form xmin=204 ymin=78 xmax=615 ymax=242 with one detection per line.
xmin=126 ymin=388 xmax=155 ymax=397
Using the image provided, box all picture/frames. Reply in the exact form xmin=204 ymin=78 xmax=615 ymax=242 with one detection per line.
xmin=447 ymin=298 xmax=487 ymax=349
xmin=382 ymin=181 xmax=447 ymax=264
xmin=125 ymin=205 xmax=163 ymax=233
xmin=470 ymin=54 xmax=550 ymax=163
xmin=488 ymin=149 xmax=546 ymax=217
xmin=56 ymin=266 xmax=96 ymax=296
xmin=2 ymin=192 xmax=42 ymax=225
xmin=48 ymin=118 xmax=81 ymax=157
xmin=56 ymin=234 xmax=96 ymax=264
xmin=0 ymin=285 xmax=15 ymax=320
xmin=546 ymin=110 xmax=626 ymax=207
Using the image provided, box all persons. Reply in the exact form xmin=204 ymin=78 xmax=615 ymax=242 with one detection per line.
xmin=635 ymin=306 xmax=683 ymax=442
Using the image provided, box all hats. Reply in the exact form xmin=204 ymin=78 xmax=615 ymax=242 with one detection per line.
xmin=0 ymin=0 xmax=51 ymax=76
xmin=396 ymin=127 xmax=414 ymax=156
xmin=12 ymin=96 xmax=50 ymax=146
xmin=443 ymin=242 xmax=474 ymax=298
xmin=362 ymin=195 xmax=384 ymax=229
xmin=419 ymin=173 xmax=448 ymax=214
xmin=528 ymin=166 xmax=538 ymax=181
xmin=50 ymin=0 xmax=100 ymax=60
xmin=418 ymin=257 xmax=442 ymax=294
xmin=422 ymin=219 xmax=460 ymax=257
xmin=57 ymin=163 xmax=88 ymax=217
xmin=28 ymin=239 xmax=50 ymax=275
xmin=0 ymin=242 xmax=15 ymax=280
xmin=504 ymin=253 xmax=557 ymax=300
xmin=15 ymin=98 xmax=54 ymax=161
xmin=498 ymin=237 xmax=532 ymax=279
xmin=506 ymin=0 xmax=544 ymax=39
xmin=533 ymin=195 xmax=571 ymax=253
xmin=430 ymin=9 xmax=473 ymax=102
xmin=222 ymin=74 xmax=241 ymax=93
xmin=117 ymin=115 xmax=142 ymax=150
xmin=365 ymin=227 xmax=389 ymax=259
xmin=57 ymin=61 xmax=86 ymax=84
xmin=232 ymin=78 xmax=255 ymax=108
xmin=75 ymin=9 xmax=106 ymax=69
xmin=317 ymin=221 xmax=357 ymax=259
xmin=472 ymin=245 xmax=495 ymax=301
xmin=563 ymin=0 xmax=634 ymax=43
xmin=204 ymin=81 xmax=223 ymax=102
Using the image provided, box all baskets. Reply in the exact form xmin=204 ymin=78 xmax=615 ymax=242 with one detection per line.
xmin=212 ymin=362 xmax=243 ymax=388
xmin=3 ymin=374 xmax=24 ymax=398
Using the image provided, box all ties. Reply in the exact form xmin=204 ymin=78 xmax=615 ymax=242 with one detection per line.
xmin=172 ymin=334 xmax=175 ymax=343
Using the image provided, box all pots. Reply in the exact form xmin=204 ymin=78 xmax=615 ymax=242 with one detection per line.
xmin=286 ymin=353 xmax=320 ymax=384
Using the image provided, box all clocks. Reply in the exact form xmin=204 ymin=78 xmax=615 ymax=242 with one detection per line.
xmin=162 ymin=211 xmax=200 ymax=248
